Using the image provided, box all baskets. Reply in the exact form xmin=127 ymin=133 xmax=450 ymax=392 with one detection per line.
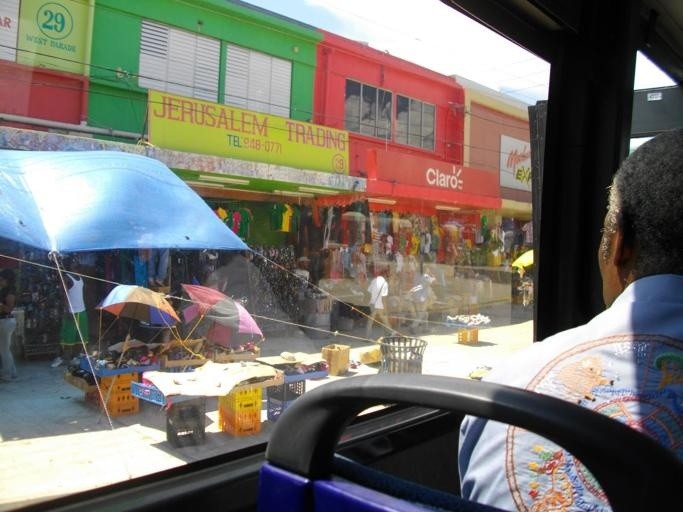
xmin=217 ymin=386 xmax=262 ymax=437
xmin=102 ymin=372 xmax=139 ymax=416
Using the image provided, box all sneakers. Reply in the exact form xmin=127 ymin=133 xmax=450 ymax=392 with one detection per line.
xmin=407 ymin=326 xmax=430 ymax=336
xmin=51 ymin=357 xmax=63 ymax=367
xmin=71 ymin=357 xmax=80 ymax=364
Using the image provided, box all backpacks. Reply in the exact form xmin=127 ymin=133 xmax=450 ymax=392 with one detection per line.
xmin=405 ymin=285 xmax=425 ymax=303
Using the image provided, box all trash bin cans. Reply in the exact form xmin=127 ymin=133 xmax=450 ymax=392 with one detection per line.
xmin=378 ymin=337 xmax=428 ymax=375
xmin=304 ymin=289 xmax=332 ymax=339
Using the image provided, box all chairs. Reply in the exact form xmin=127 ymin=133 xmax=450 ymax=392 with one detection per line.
xmin=259 ymin=375 xmax=683 ymax=511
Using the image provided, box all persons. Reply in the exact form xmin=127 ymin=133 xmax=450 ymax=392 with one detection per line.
xmin=407 ymin=264 xmax=440 ymax=335
xmin=456 ymin=127 xmax=681 ymax=511
xmin=56 ymin=257 xmax=89 ymax=368
xmin=363 ymin=269 xmax=398 ymax=341
xmin=0 ymin=269 xmax=20 ymax=384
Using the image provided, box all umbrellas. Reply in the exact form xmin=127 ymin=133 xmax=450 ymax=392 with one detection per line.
xmin=95 ymin=283 xmax=179 ymax=330
xmin=179 ymin=283 xmax=262 ymax=348
xmin=511 ymin=247 xmax=534 ymax=268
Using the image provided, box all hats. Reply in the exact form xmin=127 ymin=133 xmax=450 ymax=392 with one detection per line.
xmin=297 ymin=257 xmax=311 ymax=262
xmin=422 ymin=274 xmax=434 ymax=284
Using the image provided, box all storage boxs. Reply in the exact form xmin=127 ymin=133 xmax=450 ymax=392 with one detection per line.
xmin=321 ymin=343 xmax=351 ymax=376
